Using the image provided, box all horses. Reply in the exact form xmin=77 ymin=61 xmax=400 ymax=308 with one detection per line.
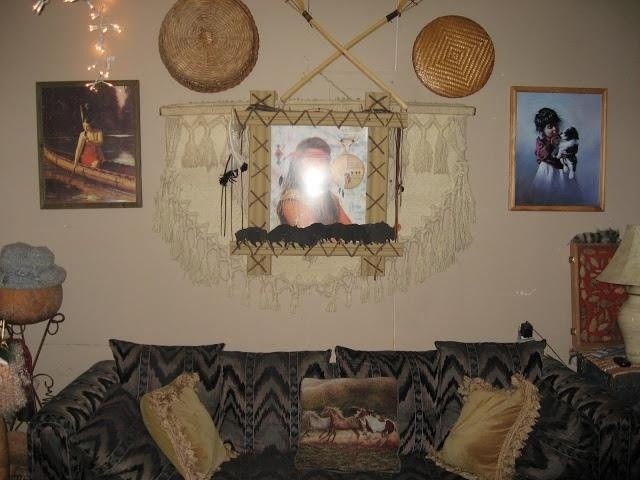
xmin=354 ymin=407 xmax=398 ymax=447
xmin=320 ymin=406 xmax=361 ymax=443
xmin=300 ymin=410 xmax=331 ymax=441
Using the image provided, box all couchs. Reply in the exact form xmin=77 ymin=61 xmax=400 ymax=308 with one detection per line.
xmin=25 ymin=339 xmax=636 ymax=478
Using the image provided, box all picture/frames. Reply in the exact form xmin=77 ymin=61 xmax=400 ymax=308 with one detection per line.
xmin=505 ymin=83 xmax=610 ymax=214
xmin=228 ymin=90 xmax=408 ymax=278
xmin=34 ymin=80 xmax=144 ymax=211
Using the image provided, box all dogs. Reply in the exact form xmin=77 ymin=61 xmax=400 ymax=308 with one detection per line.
xmin=555 ymin=126 xmax=580 ymax=180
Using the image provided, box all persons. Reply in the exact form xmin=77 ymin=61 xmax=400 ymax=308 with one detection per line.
xmin=276 ymin=137 xmax=352 ymax=227
xmin=531 ymin=107 xmax=584 ymax=203
xmin=72 ymin=113 xmax=103 ymax=168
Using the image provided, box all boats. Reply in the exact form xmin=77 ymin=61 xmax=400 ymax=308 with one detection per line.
xmin=42 ymin=145 xmax=137 ymax=194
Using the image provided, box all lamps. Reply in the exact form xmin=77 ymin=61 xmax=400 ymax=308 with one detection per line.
xmin=597 ymin=223 xmax=640 ymax=296
